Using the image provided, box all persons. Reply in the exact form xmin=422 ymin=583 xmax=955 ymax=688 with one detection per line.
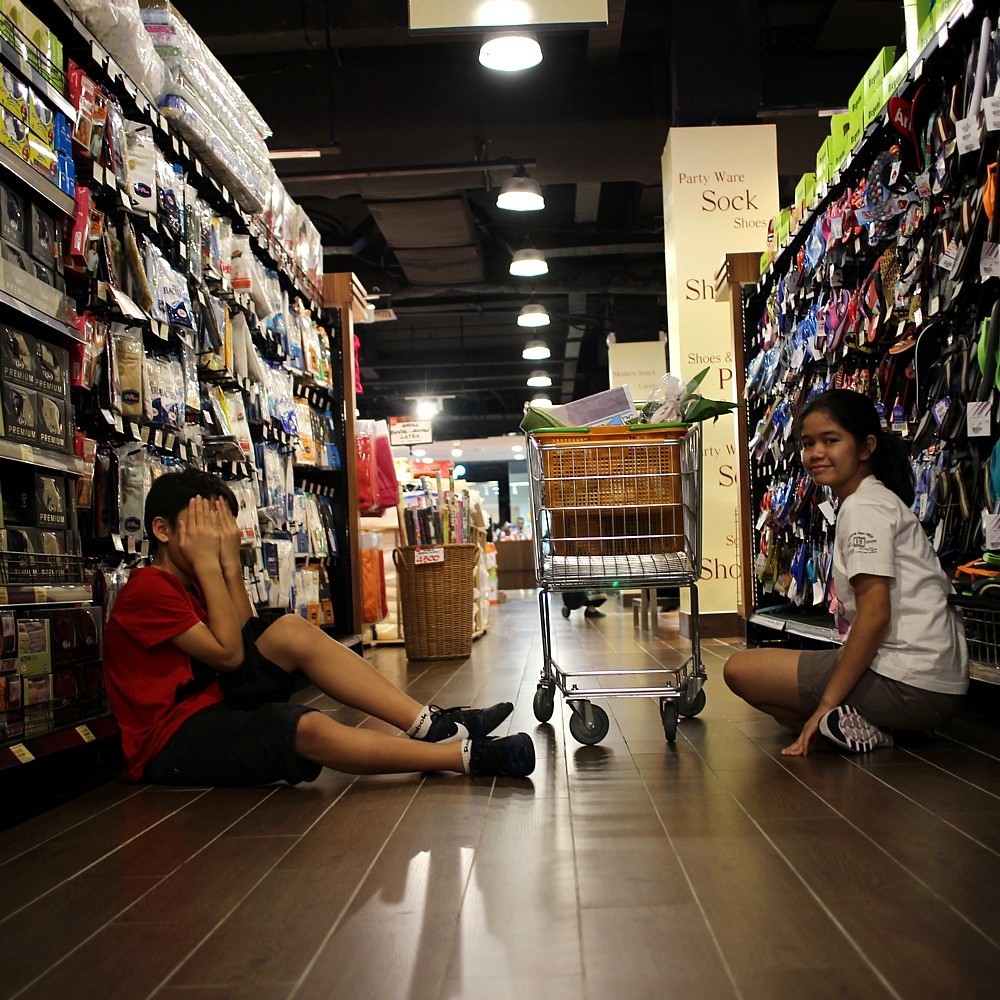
xmin=496 ymin=521 xmax=508 ymax=537
xmin=512 ymin=517 xmax=529 ymax=534
xmin=723 ymin=389 xmax=969 ymax=756
xmin=561 ymin=590 xmax=607 ymax=619
xmin=101 ymin=469 xmax=533 ymax=782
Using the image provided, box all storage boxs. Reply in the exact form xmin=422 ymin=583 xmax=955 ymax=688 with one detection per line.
xmin=0 ymin=181 xmax=67 ymax=293
xmin=0 ymin=0 xmax=66 ymax=96
xmin=0 ymin=467 xmax=75 ymax=584
xmin=0 ymin=62 xmax=76 ymax=198
xmin=0 ymin=607 xmax=105 ymax=742
xmin=253 ymin=172 xmax=324 ymax=287
xmin=565 ymin=385 xmax=637 ymax=428
xmin=760 ymin=0 xmax=955 ymax=275
xmin=0 ymin=324 xmax=72 ymax=453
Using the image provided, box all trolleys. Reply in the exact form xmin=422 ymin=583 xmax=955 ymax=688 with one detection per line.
xmin=523 ymin=417 xmax=709 ymax=744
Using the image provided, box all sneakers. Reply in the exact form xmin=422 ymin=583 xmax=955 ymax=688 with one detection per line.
xmin=407 ymin=702 xmax=514 ymax=743
xmin=469 ymin=732 xmax=535 ymax=780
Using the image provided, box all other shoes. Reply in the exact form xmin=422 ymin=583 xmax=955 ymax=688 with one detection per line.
xmin=562 ymin=605 xmax=570 ymax=618
xmin=584 ymin=608 xmax=606 ymax=618
xmin=819 ymin=705 xmax=895 ymax=752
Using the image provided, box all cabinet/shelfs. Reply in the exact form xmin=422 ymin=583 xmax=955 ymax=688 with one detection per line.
xmin=359 ymin=524 xmax=483 ymax=647
xmin=0 ymin=1 xmax=367 ymax=828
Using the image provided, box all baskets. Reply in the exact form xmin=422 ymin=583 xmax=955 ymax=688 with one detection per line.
xmin=551 ymin=505 xmax=685 ymax=556
xmin=393 ymin=543 xmax=483 ymax=662
xmin=528 ymin=423 xmax=690 ymax=516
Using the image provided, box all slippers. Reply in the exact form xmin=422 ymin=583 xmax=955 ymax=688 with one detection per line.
xmin=746 ymin=17 xmax=1000 ymax=668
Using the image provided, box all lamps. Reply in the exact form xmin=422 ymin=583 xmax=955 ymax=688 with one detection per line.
xmin=523 ymin=328 xmax=551 ymax=359
xmin=509 ymin=235 xmax=549 ymax=277
xmin=268 ymin=0 xmax=341 ymax=159
xmin=496 ymin=165 xmax=545 ymax=211
xmin=530 ymin=393 xmax=552 ymax=407
xmin=516 ymin=283 xmax=551 ymax=327
xmin=478 ymin=30 xmax=543 ymax=72
xmin=527 ymin=370 xmax=552 ymax=387
